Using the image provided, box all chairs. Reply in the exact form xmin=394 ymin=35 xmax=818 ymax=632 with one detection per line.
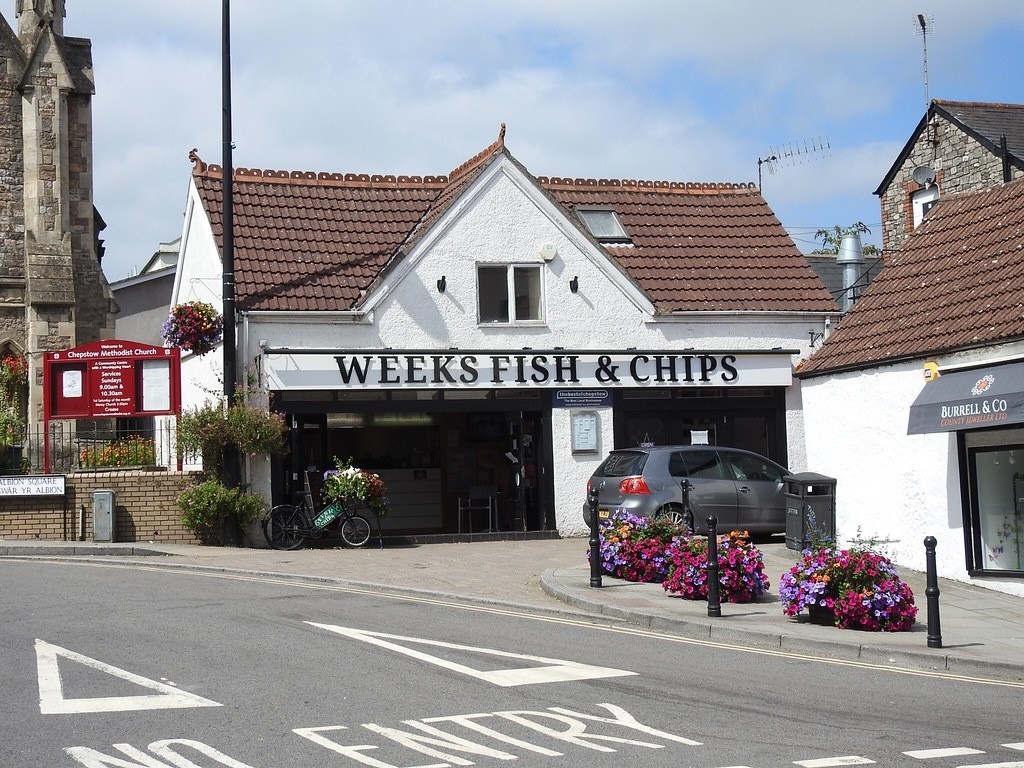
xmin=456 ymin=465 xmax=505 ymax=534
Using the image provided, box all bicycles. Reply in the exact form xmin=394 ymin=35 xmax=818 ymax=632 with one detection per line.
xmin=261 ymin=478 xmax=371 ymax=550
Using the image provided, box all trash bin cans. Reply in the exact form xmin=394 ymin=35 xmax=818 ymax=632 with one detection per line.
xmin=783 ymin=472 xmax=838 ymax=553
xmin=0 ymin=445 xmax=24 ymax=476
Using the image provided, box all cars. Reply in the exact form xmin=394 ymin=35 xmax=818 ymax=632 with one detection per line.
xmin=583 ymin=444 xmax=796 ymax=536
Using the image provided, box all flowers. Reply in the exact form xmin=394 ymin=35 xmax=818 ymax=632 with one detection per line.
xmin=175 ymin=475 xmax=268 ymax=526
xmin=323 ymin=466 xmax=391 ymax=516
xmin=586 ymin=503 xmax=921 ymax=635
xmin=159 ymin=300 xmax=223 ymax=355
xmin=169 ymin=372 xmax=289 ymax=458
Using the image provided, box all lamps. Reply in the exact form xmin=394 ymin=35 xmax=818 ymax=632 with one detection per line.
xmin=540 ymin=244 xmax=557 ymax=261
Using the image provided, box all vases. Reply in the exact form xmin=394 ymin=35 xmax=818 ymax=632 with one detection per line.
xmin=810 ymin=598 xmax=842 ymax=628
xmin=344 ymin=491 xmax=377 ymax=509
